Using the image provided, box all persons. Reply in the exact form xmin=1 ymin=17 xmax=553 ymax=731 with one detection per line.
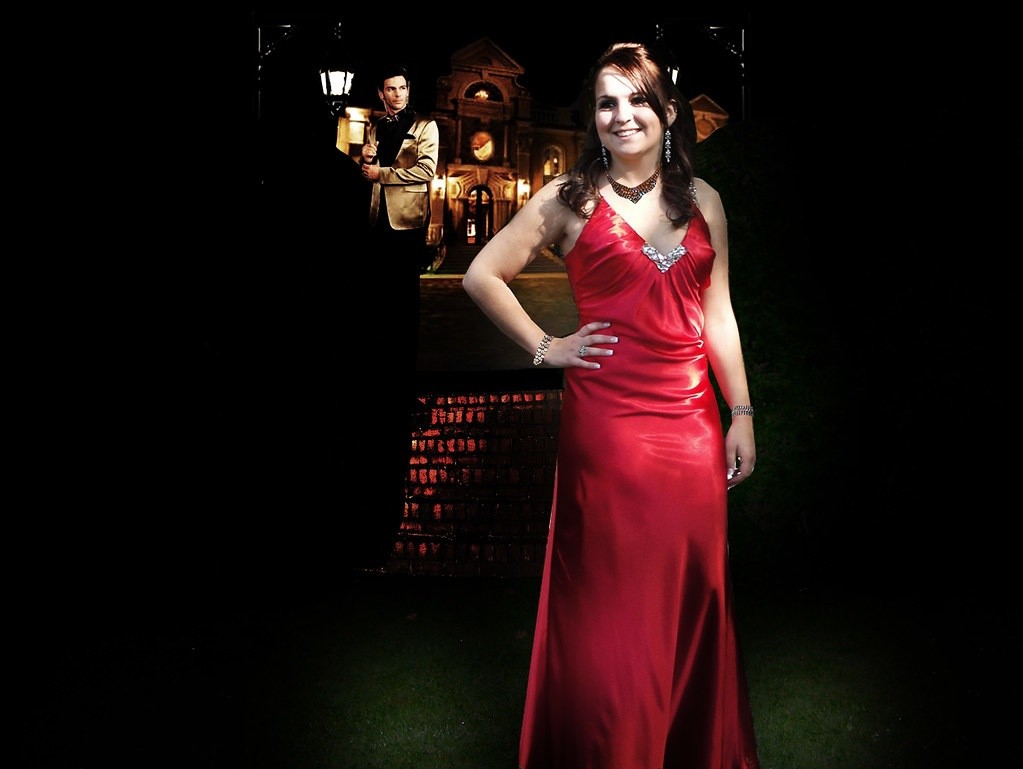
xmin=461 ymin=37 xmax=756 ymax=769
xmin=361 ymin=64 xmax=440 ymax=304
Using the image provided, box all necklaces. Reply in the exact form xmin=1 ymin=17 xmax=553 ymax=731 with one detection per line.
xmin=605 ymin=163 xmax=660 ymax=203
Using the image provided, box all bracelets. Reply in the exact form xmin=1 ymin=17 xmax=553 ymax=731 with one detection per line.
xmin=532 ymin=333 xmax=554 ymax=366
xmin=732 ymin=405 xmax=754 ymax=417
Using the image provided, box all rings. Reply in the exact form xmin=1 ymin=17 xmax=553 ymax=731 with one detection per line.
xmin=579 ymin=345 xmax=586 ymax=358
